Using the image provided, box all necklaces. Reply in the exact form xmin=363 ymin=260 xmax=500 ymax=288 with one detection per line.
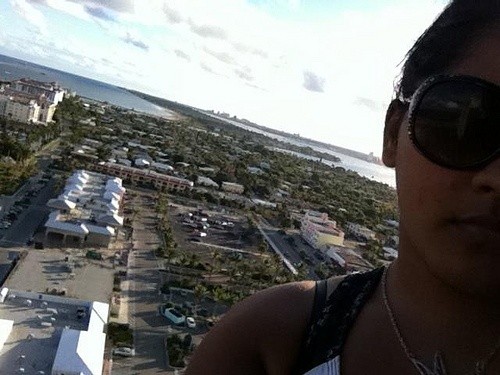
xmin=381 ymin=264 xmax=486 ymax=375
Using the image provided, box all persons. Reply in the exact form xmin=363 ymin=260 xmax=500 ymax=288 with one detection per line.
xmin=185 ymin=0 xmax=500 ymax=375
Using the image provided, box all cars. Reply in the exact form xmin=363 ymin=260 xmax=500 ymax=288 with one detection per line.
xmin=179 ymin=212 xmax=234 ymax=243
xmin=187 ymin=317 xmax=198 ymax=328
xmin=113 ymin=347 xmax=135 ymax=356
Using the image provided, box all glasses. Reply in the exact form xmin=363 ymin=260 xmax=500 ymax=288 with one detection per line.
xmin=398 ymin=73 xmax=500 ymax=172
